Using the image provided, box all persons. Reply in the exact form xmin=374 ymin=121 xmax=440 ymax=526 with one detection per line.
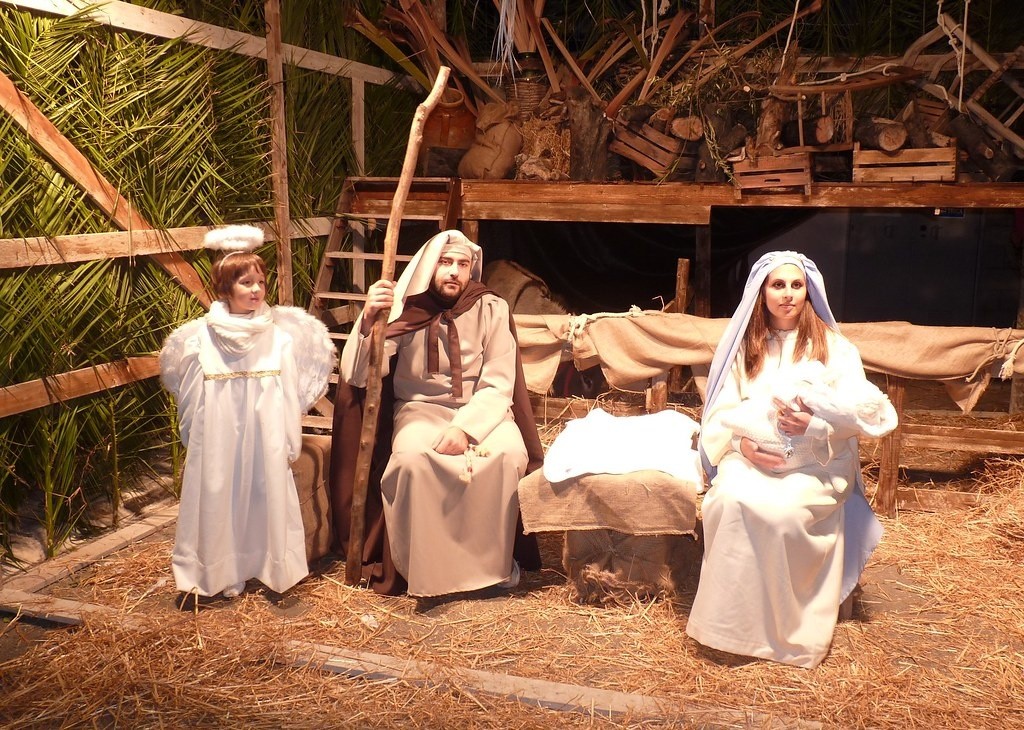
xmin=340 ymin=229 xmax=545 ymax=599
xmin=718 ymin=375 xmax=900 ymax=472
xmin=158 ymin=225 xmax=342 ymax=598
xmin=683 ymin=248 xmax=882 ymax=673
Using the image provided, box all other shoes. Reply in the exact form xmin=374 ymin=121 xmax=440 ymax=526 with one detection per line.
xmin=497 ymin=557 xmax=519 ymax=588
xmin=222 ymin=579 xmax=246 ymax=597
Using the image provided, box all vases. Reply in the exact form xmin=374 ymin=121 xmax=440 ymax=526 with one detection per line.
xmin=503 ymin=50 xmax=549 ymax=119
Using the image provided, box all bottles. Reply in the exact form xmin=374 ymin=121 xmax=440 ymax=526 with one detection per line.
xmin=425 ymin=91 xmax=474 ymax=149
xmin=511 ymin=52 xmax=547 ymax=84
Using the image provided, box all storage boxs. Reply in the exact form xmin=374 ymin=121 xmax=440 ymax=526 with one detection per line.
xmin=916 ymin=96 xmax=950 ymax=134
xmin=852 ymin=137 xmax=961 ymax=183
xmin=732 ymin=153 xmax=814 ymax=199
xmin=608 ymin=122 xmax=698 ymax=182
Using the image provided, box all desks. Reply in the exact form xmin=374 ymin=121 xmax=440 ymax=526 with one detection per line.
xmin=516 ymin=408 xmax=705 ymax=600
